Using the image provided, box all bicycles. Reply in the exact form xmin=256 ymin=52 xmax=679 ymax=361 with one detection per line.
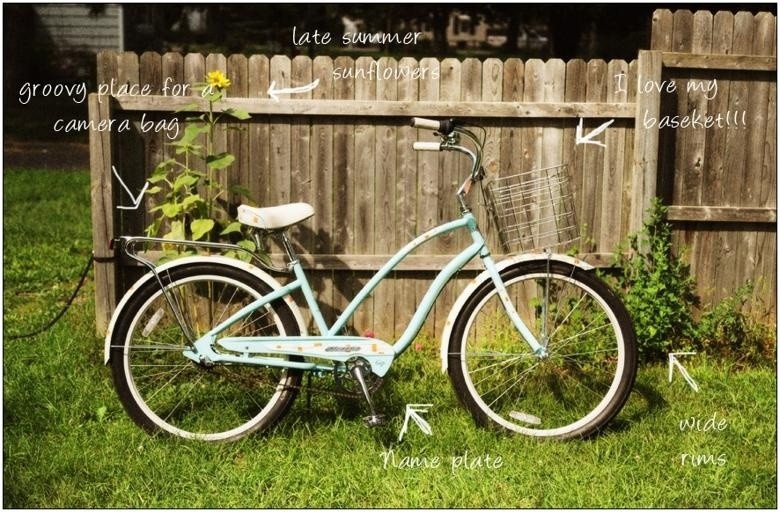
xmin=99 ymin=113 xmax=640 ymax=443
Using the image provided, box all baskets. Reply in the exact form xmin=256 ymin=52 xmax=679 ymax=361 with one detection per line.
xmin=481 ymin=161 xmax=583 ymax=254
